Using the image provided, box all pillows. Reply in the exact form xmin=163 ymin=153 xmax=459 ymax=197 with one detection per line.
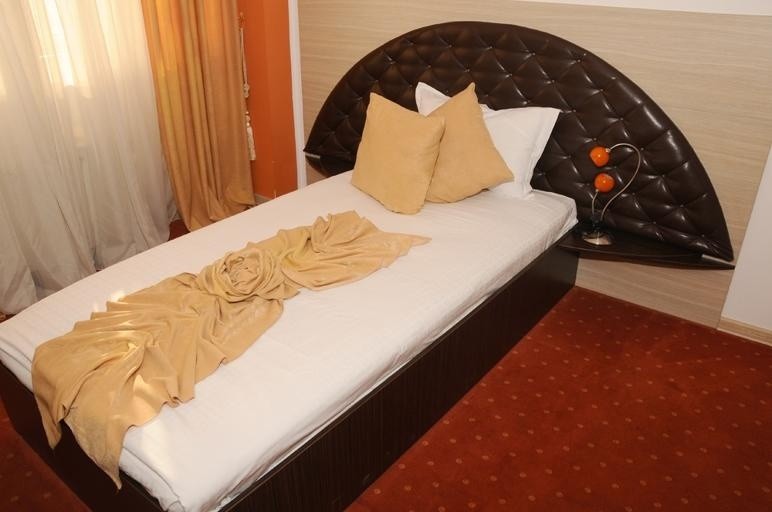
xmin=421 ymin=81 xmax=516 ymax=204
xmin=354 ymin=92 xmax=447 ymax=216
xmin=414 ymin=81 xmax=563 ymax=195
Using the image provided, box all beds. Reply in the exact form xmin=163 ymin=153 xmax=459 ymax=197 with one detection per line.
xmin=0 ymin=170 xmax=580 ymax=512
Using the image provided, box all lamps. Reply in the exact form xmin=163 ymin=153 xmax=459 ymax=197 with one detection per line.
xmin=582 ymin=142 xmax=642 ymax=245
